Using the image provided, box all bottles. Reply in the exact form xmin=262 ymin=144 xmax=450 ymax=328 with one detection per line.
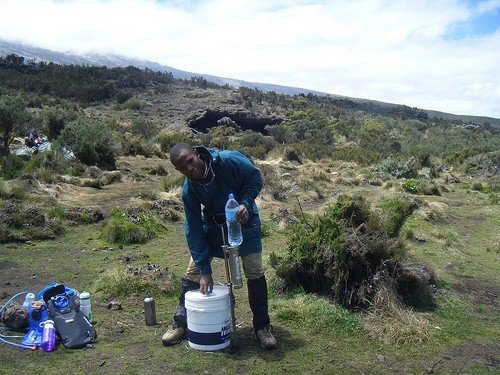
xmin=224 ymin=192 xmax=242 ymax=246
xmin=22 ymin=289 xmax=36 ymax=306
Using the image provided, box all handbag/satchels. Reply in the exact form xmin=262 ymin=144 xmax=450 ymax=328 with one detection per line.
xmin=202 ymin=206 xmax=227 ymax=225
xmin=47 ymin=294 xmax=97 ymax=349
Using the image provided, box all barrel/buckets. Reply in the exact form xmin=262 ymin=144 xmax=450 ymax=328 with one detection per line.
xmin=184 ymin=285 xmax=232 ymax=351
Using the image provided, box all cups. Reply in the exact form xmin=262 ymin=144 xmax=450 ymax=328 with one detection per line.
xmin=40 ymin=320 xmax=55 ymax=351
xmin=144 ymin=291 xmax=155 ymax=326
xmin=80 ymin=288 xmax=91 ymax=321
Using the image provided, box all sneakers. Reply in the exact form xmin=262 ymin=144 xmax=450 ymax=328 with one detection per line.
xmin=254 ymin=324 xmax=277 ymax=350
xmin=162 ymin=324 xmax=188 ymax=346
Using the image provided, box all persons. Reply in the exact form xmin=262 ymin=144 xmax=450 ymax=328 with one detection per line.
xmin=161 ymin=143 xmax=278 ymax=349
xmin=24 ymin=127 xmax=48 ymax=147
